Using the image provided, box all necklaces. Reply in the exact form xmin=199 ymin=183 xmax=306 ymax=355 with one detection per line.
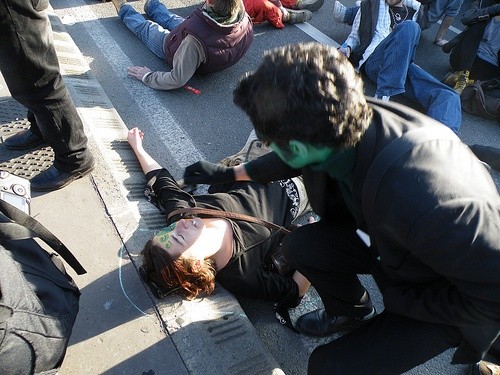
xmin=389 ymin=3 xmax=409 ymax=29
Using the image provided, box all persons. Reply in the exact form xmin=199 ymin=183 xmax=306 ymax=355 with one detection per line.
xmin=110 ymin=0 xmax=255 ymax=92
xmin=126 ymin=127 xmax=312 ymax=312
xmin=0 ymin=0 xmax=96 ymax=192
xmin=180 ymin=40 xmax=500 ymax=375
xmin=332 ymin=0 xmax=500 ymax=168
xmin=243 ymin=0 xmax=324 ymax=30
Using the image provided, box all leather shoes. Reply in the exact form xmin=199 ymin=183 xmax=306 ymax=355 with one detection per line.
xmin=28 ymin=157 xmax=95 ymax=191
xmin=5 ymin=128 xmax=45 ymax=150
xmin=295 ymin=306 xmax=376 ymax=337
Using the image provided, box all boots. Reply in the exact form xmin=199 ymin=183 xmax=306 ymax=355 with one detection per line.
xmin=441 ymin=71 xmax=475 ymax=86
xmin=453 ymin=70 xmax=470 ymax=95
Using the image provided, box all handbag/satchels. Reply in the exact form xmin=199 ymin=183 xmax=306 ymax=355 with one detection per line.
xmin=460 ymin=87 xmax=500 ymax=120
xmin=0 ymin=210 xmax=81 ymax=374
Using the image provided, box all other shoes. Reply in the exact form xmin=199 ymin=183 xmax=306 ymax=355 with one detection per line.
xmin=113 ymin=0 xmax=126 ymax=13
xmin=297 ymin=0 xmax=324 ymax=12
xmin=287 ymin=9 xmax=313 ymax=24
xmin=434 ymin=39 xmax=449 ymax=46
xmin=333 ymin=1 xmax=346 ymax=23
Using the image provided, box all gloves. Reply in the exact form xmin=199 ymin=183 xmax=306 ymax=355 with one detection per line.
xmin=182 ymin=160 xmax=235 ymax=185
